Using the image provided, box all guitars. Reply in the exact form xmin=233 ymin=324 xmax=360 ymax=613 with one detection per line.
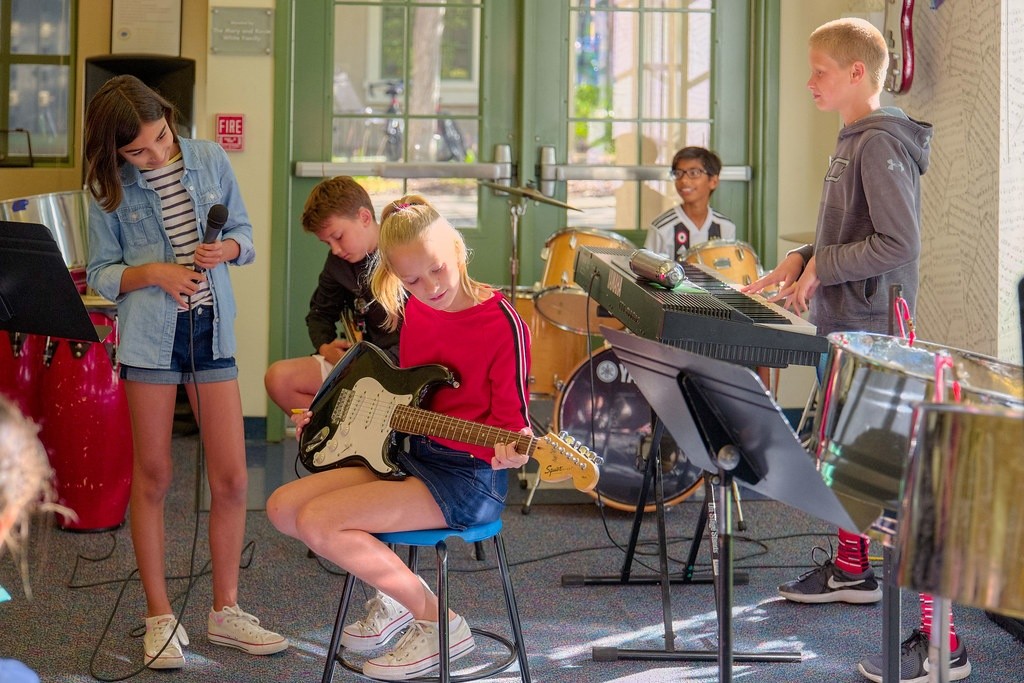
xmin=340 ymin=306 xmax=363 ymax=347
xmin=298 ymin=340 xmax=604 ymax=493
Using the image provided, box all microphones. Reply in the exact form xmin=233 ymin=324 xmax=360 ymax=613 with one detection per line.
xmin=193 ymin=204 xmax=229 ymax=283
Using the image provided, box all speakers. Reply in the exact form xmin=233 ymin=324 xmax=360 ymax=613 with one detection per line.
xmin=85 ymin=54 xmax=196 ymax=138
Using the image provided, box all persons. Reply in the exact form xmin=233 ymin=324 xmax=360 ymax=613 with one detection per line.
xmin=266 ymin=196 xmax=532 ymax=681
xmin=0 ymin=397 xmax=78 ymax=683
xmin=741 ymin=16 xmax=972 ymax=682
xmin=83 ymin=75 xmax=289 ymax=668
xmin=642 ymin=146 xmax=736 ymax=465
xmin=264 ymin=175 xmax=381 ymax=417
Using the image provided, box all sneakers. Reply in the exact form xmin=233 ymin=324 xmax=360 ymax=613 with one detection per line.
xmin=363 ymin=616 xmax=475 ymax=680
xmin=778 ymin=546 xmax=883 ymax=603
xmin=858 ymin=629 xmax=971 ymax=683
xmin=143 ymin=614 xmax=189 ymax=669
xmin=207 ymin=605 xmax=289 ymax=655
xmin=339 ymin=573 xmax=434 ymax=650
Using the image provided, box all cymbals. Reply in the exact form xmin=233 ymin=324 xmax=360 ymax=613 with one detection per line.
xmin=486 ymin=182 xmax=585 ymax=213
xmin=779 ymin=231 xmax=816 ymax=243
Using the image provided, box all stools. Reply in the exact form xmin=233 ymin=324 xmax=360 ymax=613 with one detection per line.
xmin=321 ymin=515 xmax=531 ymax=683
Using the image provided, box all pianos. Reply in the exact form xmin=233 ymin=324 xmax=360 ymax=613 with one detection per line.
xmin=561 ymin=243 xmax=828 ymax=662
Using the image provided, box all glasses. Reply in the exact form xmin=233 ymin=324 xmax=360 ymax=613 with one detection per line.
xmin=669 ymin=168 xmax=713 ymax=181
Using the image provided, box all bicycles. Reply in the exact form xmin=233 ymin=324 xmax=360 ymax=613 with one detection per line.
xmin=435 ymin=97 xmax=466 ymax=165
xmin=369 ymin=78 xmax=403 ymax=165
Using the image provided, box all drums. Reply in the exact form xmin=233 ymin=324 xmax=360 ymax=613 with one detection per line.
xmin=806 ymin=331 xmax=1024 ymax=513
xmin=888 ymin=399 xmax=1024 ymax=620
xmin=553 ymin=344 xmax=705 ymax=512
xmin=761 ymin=270 xmax=810 ymax=316
xmin=38 ymin=295 xmax=134 ymax=534
xmin=676 ymin=238 xmax=764 ymax=294
xmin=0 ymin=330 xmax=39 ymax=424
xmin=491 ymin=284 xmax=589 ymax=401
xmin=67 ymin=264 xmax=86 ymax=295
xmin=533 ymin=227 xmax=637 ymax=337
